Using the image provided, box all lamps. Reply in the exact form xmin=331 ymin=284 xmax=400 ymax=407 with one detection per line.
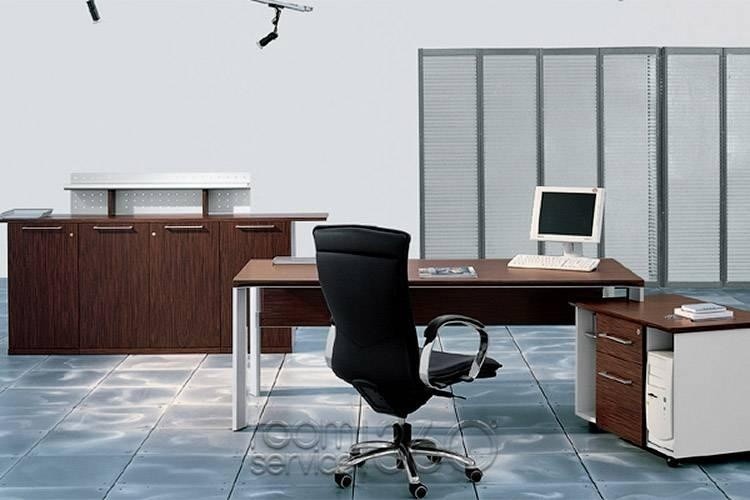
xmin=250 ymin=1 xmax=313 ymax=50
xmin=85 ymin=0 xmax=103 ymax=24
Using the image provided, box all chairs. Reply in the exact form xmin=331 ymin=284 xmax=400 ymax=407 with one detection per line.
xmin=311 ymin=224 xmax=504 ymax=500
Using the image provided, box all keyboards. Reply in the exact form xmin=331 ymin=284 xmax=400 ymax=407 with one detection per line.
xmin=507 ymin=253 xmax=600 ymax=272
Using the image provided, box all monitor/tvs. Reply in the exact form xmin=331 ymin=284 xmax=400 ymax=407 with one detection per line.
xmin=530 ymin=187 xmax=606 ymax=255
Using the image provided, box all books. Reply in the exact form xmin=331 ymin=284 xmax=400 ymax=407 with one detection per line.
xmin=417 ymin=264 xmax=479 ymax=279
xmin=0 ymin=207 xmax=54 ymax=220
xmin=662 ymin=300 xmax=735 ymax=323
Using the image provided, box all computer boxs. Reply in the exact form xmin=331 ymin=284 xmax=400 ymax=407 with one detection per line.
xmin=646 ymin=349 xmax=674 ymax=453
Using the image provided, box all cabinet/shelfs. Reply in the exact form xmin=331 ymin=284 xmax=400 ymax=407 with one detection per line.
xmin=144 ymin=211 xmax=223 ymax=357
xmin=0 ymin=212 xmax=81 ymax=358
xmin=212 ymin=209 xmax=299 ymax=358
xmin=559 ymin=287 xmax=750 ymax=467
xmin=73 ymin=212 xmax=155 ymax=357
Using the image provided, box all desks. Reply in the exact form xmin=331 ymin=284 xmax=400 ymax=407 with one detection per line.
xmin=226 ymin=254 xmax=652 ymax=447
